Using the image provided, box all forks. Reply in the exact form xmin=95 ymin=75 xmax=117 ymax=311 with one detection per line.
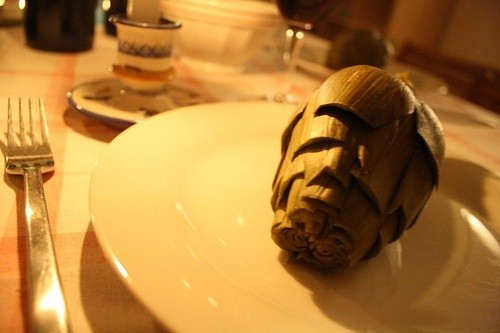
xmin=5 ymin=94 xmax=73 ymax=333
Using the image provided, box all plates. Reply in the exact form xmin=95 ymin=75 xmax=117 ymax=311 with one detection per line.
xmin=68 ymin=77 xmax=217 ymax=130
xmin=295 ymin=42 xmax=448 ymax=101
xmin=87 ymin=100 xmax=500 ymax=333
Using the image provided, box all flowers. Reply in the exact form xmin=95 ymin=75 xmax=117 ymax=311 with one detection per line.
xmin=270 ymin=65 xmax=446 ymax=274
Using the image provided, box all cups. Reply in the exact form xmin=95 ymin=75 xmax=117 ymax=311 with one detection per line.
xmin=111 ymin=18 xmax=180 ymax=91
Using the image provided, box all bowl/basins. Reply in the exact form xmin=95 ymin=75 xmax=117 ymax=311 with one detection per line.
xmin=25 ymin=0 xmax=96 ymax=52
